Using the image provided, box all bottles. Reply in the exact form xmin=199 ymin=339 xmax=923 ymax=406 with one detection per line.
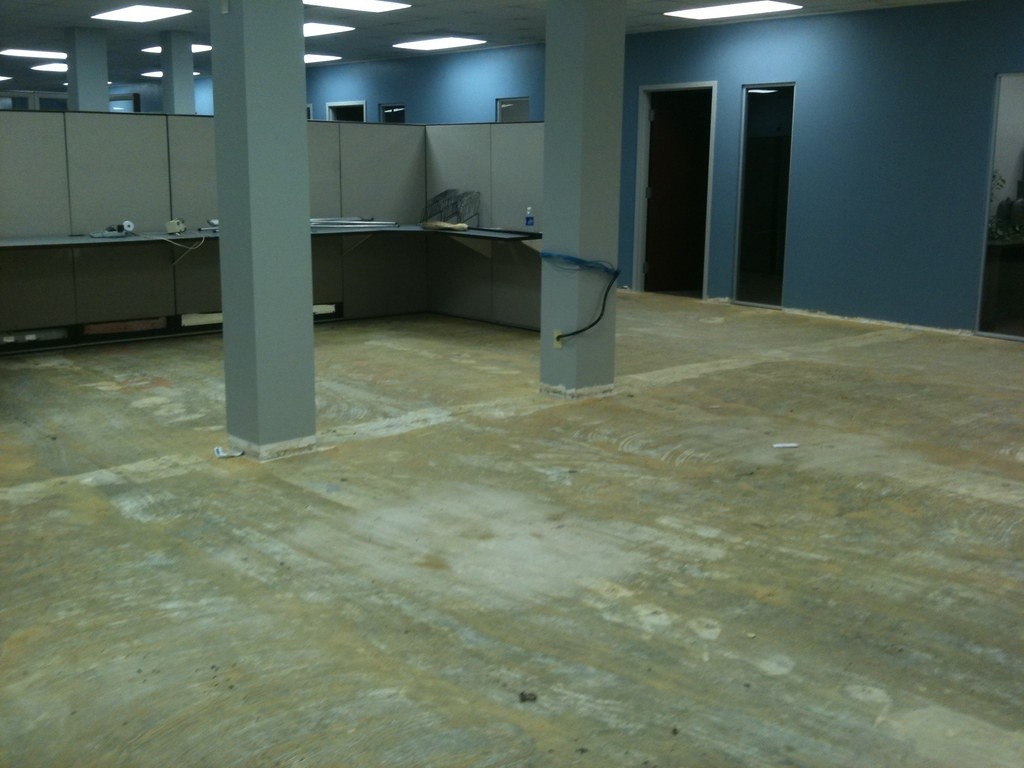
xmin=526 ymin=207 xmax=534 ymax=232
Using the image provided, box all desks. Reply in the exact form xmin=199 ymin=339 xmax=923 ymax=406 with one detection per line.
xmin=0 ymin=217 xmax=543 ymax=249
